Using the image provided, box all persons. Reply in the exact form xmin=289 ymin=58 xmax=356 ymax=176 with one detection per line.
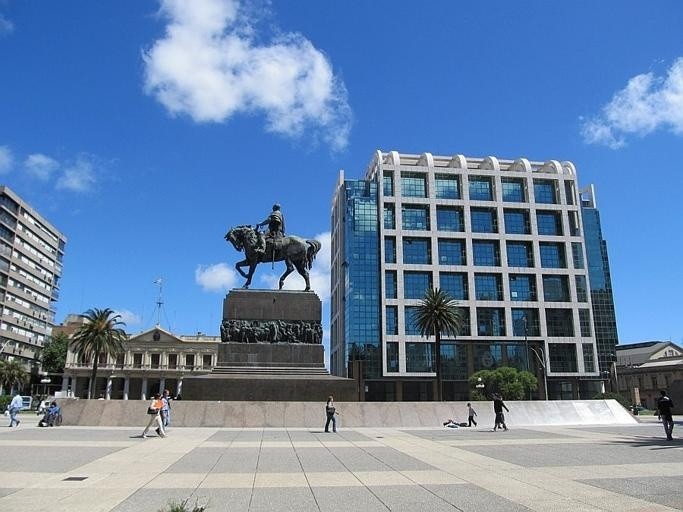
xmin=493 ymin=393 xmax=509 ymax=431
xmin=467 ymin=403 xmax=476 ymax=427
xmin=46 ymin=402 xmax=59 ymax=426
xmin=254 ymin=204 xmax=285 ymax=253
xmin=498 ymin=396 xmax=505 ymax=428
xmin=142 ymin=393 xmax=168 ymax=439
xmin=658 ymin=390 xmax=674 ymax=441
xmin=156 ymin=389 xmax=172 ymax=435
xmin=325 ymin=396 xmax=339 ymax=433
xmin=8 ymin=391 xmax=23 ymax=427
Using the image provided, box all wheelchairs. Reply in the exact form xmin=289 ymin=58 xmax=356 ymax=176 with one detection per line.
xmin=39 ymin=408 xmax=62 ymax=426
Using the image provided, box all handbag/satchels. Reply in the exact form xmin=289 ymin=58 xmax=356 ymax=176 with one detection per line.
xmin=326 ymin=406 xmax=335 ymax=414
xmin=147 ymin=407 xmax=159 ymax=414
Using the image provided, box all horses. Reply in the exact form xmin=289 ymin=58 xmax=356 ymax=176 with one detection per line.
xmin=224 ymin=224 xmax=321 ymax=292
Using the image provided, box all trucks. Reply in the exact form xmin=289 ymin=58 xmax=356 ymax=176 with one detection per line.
xmin=20 ymin=396 xmax=33 ymax=411
